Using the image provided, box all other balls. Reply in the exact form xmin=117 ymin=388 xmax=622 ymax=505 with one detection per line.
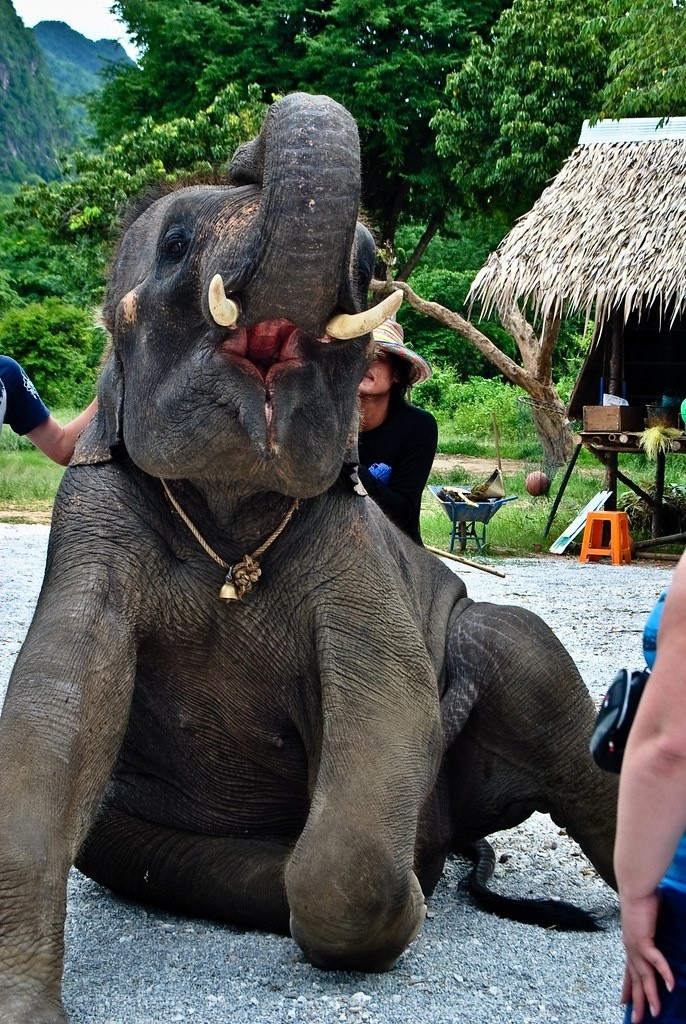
xmin=525 ymin=472 xmax=549 ymax=496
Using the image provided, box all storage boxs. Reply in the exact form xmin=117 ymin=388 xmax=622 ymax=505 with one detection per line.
xmin=583 ymin=405 xmax=645 ymax=432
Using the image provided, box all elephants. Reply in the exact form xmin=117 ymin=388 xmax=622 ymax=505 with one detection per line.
xmin=0 ymin=92 xmax=624 ymax=1024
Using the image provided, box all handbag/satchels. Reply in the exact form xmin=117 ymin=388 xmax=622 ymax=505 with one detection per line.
xmin=589 ymin=667 xmax=650 ymax=774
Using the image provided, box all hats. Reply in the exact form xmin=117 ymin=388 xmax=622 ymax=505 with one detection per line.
xmin=371 ymin=318 xmax=431 ymax=386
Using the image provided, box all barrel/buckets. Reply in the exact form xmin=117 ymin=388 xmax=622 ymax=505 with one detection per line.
xmin=645 ymin=390 xmax=679 ymax=429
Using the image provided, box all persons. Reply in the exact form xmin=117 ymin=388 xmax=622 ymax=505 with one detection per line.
xmin=614 ymin=551 xmax=686 ymax=1024
xmin=0 ymin=354 xmax=99 ymax=467
xmin=356 ymin=319 xmax=439 ymax=548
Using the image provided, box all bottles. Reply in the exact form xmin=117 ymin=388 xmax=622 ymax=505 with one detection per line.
xmin=368 ymin=463 xmax=392 ymax=485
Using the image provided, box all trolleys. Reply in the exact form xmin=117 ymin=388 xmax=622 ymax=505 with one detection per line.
xmin=428 ymin=485 xmax=518 ymax=557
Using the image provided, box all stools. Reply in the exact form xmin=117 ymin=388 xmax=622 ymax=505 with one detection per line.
xmin=580 ymin=510 xmax=631 ymax=567
